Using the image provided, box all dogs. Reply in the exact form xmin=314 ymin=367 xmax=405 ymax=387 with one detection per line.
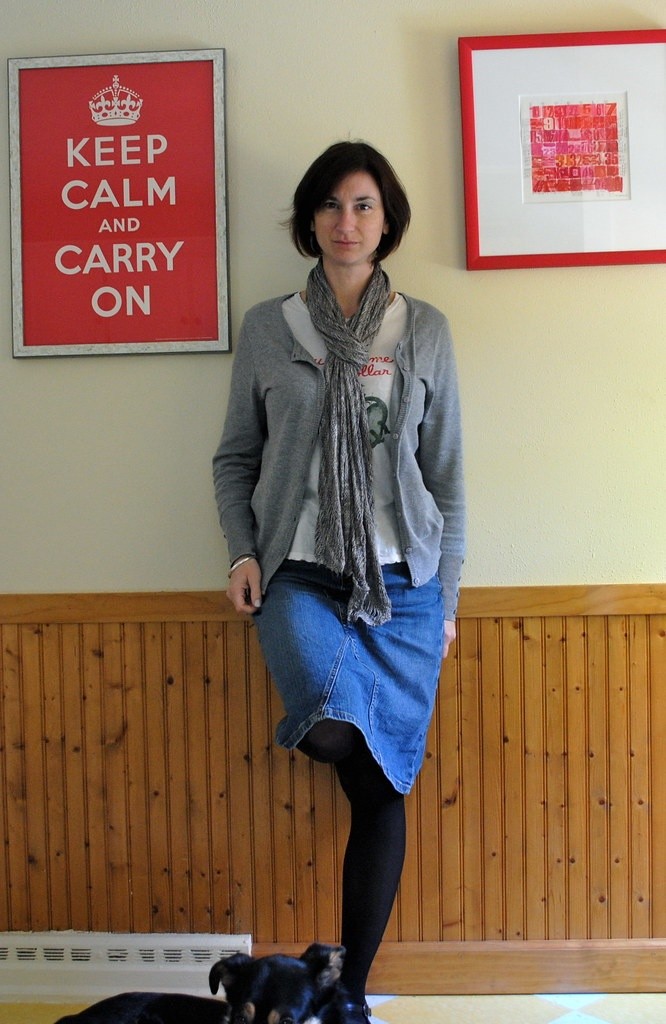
xmin=55 ymin=943 xmax=352 ymax=1024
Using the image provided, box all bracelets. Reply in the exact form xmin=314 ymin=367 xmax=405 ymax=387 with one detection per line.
xmin=228 ymin=556 xmax=255 ymax=579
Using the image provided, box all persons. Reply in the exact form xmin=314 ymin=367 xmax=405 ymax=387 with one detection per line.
xmin=212 ymin=140 xmax=466 ymax=1023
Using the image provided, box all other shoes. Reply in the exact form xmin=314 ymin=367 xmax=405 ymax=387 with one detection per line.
xmin=313 ymin=981 xmax=371 ymax=1024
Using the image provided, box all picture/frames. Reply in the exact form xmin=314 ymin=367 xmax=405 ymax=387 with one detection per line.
xmin=456 ymin=29 xmax=666 ymax=272
xmin=5 ymin=47 xmax=233 ymax=360
